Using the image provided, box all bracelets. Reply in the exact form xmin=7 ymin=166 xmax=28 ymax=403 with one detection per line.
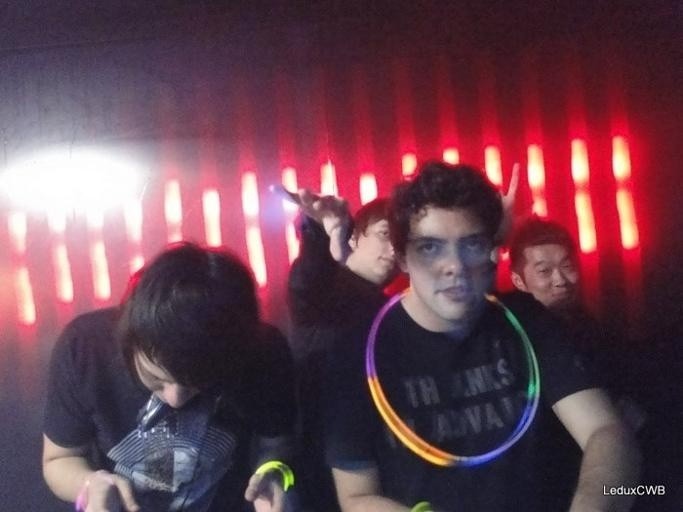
xmin=73 ymin=467 xmax=108 ymax=512
xmin=410 ymin=499 xmax=433 ymax=512
xmin=254 ymin=458 xmax=296 ymax=492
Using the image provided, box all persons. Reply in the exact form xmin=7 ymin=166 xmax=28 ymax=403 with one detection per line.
xmin=314 ymin=155 xmax=646 ymax=511
xmin=37 ymin=238 xmax=307 ymax=512
xmin=264 ymin=178 xmax=398 ymax=512
xmin=480 ymin=160 xmax=662 ymax=442
